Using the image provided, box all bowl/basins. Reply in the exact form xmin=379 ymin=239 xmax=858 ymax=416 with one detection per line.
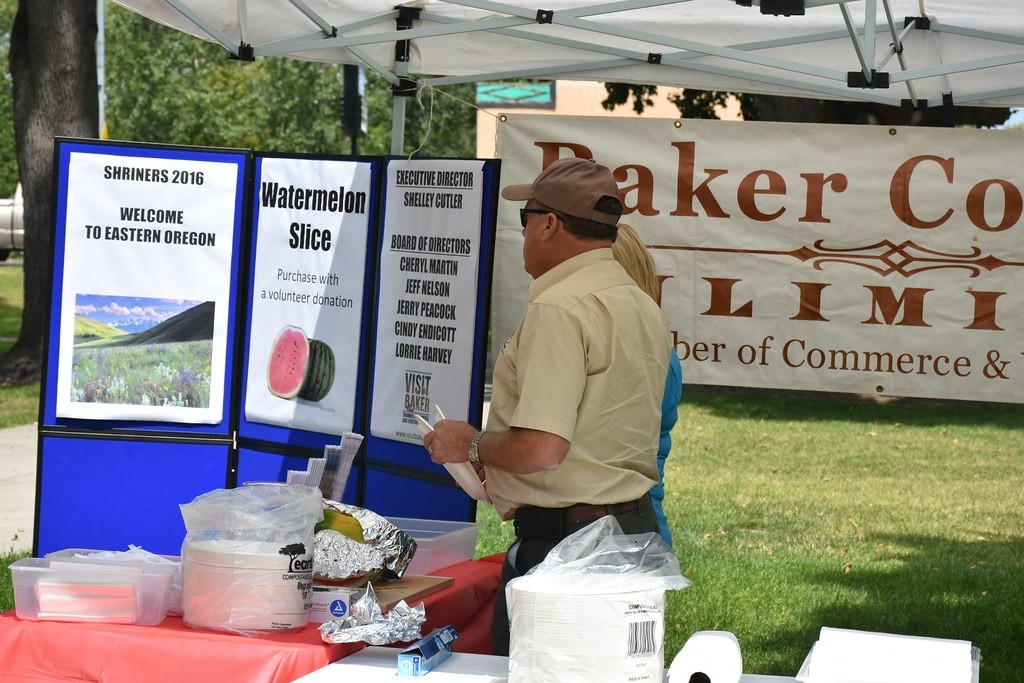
xmin=43 ymin=548 xmax=183 ymax=618
xmin=7 ymin=557 xmax=179 ymax=627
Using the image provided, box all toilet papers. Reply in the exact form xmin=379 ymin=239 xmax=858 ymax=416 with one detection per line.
xmin=665 ymin=630 xmax=744 ymax=683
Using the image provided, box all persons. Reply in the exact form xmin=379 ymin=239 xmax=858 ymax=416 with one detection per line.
xmin=423 ymin=158 xmax=670 ymax=658
xmin=612 ymin=224 xmax=683 ymax=546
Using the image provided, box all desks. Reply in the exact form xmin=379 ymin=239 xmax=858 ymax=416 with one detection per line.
xmin=284 ymin=644 xmax=802 ymax=683
xmin=1 ymin=553 xmax=504 ymax=683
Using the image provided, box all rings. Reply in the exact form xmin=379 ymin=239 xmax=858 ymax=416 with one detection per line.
xmin=428 ymin=444 xmax=434 ymax=453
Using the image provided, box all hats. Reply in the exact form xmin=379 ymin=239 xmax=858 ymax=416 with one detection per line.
xmin=501 ymin=157 xmax=622 ymax=227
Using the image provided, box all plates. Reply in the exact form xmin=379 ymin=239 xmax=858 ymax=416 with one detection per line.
xmin=508 ymin=576 xmax=663 ymax=683
xmin=183 ymin=539 xmax=315 ymax=630
xmin=412 ymin=404 xmax=494 ymax=507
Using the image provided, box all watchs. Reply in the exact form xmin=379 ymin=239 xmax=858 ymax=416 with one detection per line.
xmin=469 ymin=431 xmax=487 ymax=464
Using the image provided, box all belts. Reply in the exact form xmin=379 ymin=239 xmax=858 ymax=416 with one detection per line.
xmin=566 ymin=491 xmax=650 ymax=524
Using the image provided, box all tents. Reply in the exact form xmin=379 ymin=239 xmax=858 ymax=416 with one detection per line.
xmin=115 ymin=0 xmax=1023 ymax=153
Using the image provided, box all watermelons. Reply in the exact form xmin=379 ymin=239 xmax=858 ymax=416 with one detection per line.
xmin=314 ymin=510 xmax=387 ymax=589
xmin=266 ymin=325 xmax=335 ymax=402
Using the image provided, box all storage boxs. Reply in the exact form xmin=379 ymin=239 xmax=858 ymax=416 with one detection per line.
xmin=309 ymin=582 xmax=368 ymax=625
xmin=395 ymin=625 xmax=460 ymax=678
xmin=6 ymin=557 xmax=178 ymax=626
xmin=43 ymin=547 xmax=183 ymax=618
xmin=383 ymin=516 xmax=480 ymax=576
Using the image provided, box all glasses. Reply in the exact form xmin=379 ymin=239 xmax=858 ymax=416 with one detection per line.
xmin=519 ymin=204 xmax=566 ymax=227
